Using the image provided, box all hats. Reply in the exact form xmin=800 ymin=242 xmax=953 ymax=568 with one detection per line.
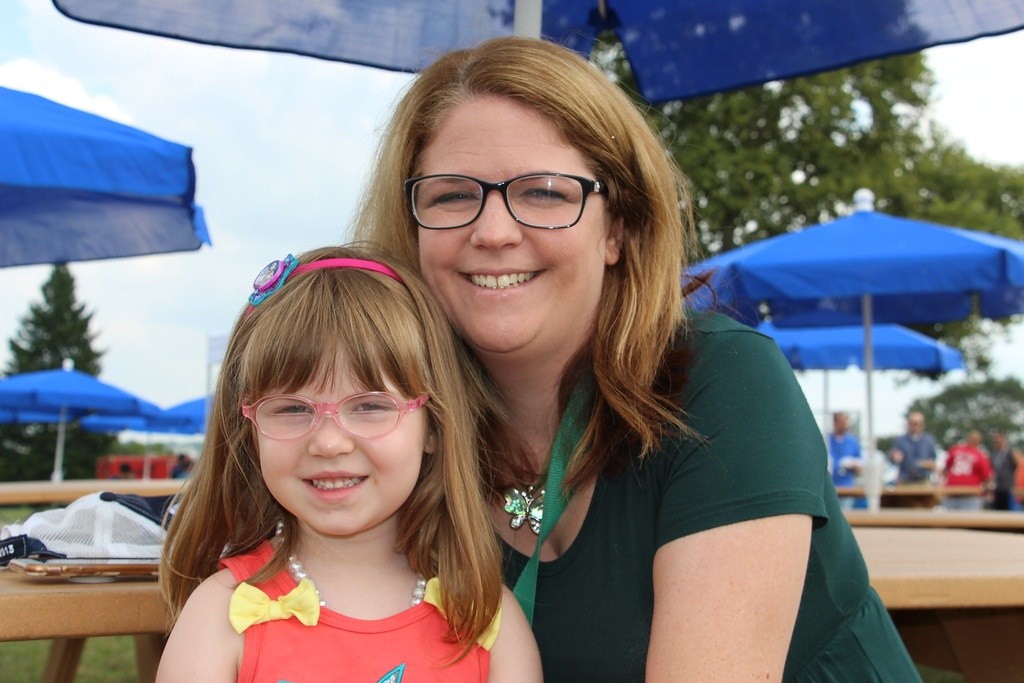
xmin=21 ymin=492 xmax=169 ymax=562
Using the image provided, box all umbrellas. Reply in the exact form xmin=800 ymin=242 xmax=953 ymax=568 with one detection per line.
xmin=0 ymin=85 xmax=212 ymax=269
xmin=155 ymin=394 xmax=214 ymax=428
xmin=0 ymin=371 xmax=160 ymax=483
xmin=83 ymin=409 xmax=200 ymax=481
xmin=678 ymin=186 xmax=1024 ymax=509
xmin=754 ymin=315 xmax=966 ymax=432
xmin=52 ymin=0 xmax=1024 ymax=102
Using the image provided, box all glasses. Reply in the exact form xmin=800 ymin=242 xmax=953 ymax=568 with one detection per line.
xmin=242 ymin=390 xmax=431 ymax=442
xmin=404 ymin=172 xmax=607 ymax=231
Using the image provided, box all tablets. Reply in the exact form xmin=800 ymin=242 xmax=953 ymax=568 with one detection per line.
xmin=8 ymin=557 xmax=162 ymax=577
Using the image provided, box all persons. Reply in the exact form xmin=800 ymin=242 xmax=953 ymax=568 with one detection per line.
xmin=939 ymin=428 xmax=1024 ymax=512
xmin=887 ymin=410 xmax=937 ymax=487
xmin=153 ymin=242 xmax=545 ymax=683
xmin=824 ymin=410 xmax=862 ymax=509
xmin=349 ymin=38 xmax=926 ymax=683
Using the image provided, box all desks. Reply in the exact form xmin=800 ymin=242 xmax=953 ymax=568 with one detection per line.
xmin=0 ymin=476 xmax=196 ymax=507
xmin=1 ymin=565 xmax=197 ymax=683
xmin=844 ymin=482 xmax=1023 ymax=683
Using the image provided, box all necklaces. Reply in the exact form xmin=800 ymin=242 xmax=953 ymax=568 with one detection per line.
xmin=274 ymin=519 xmax=428 ymax=609
xmin=501 ymin=473 xmax=545 ymax=536
